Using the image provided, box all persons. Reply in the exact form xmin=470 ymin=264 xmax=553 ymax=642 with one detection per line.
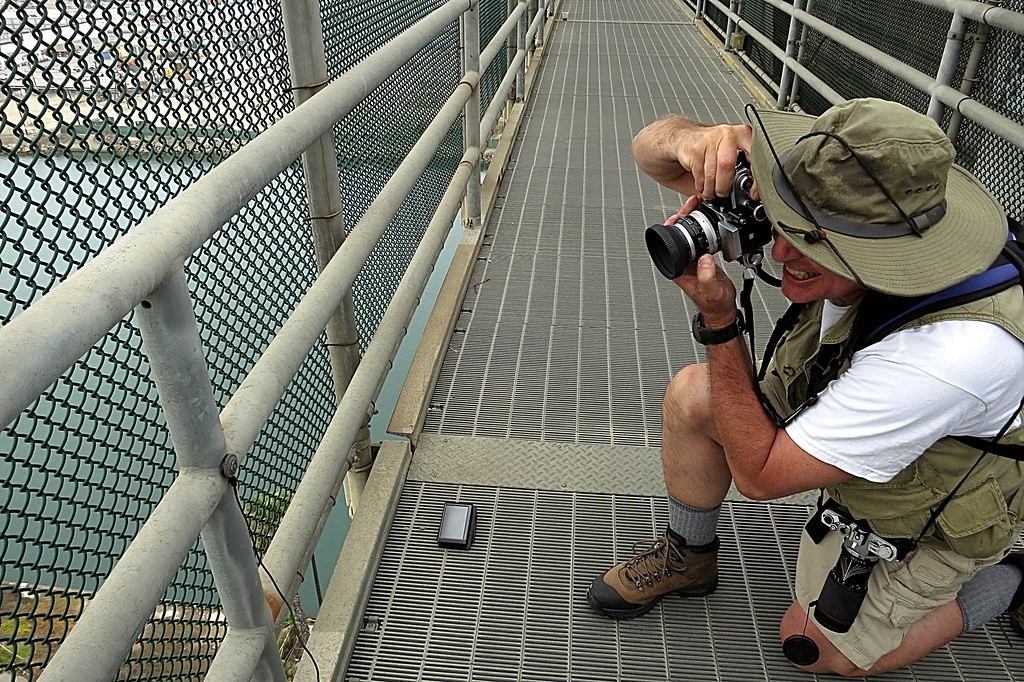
xmin=585 ymin=98 xmax=1024 ymax=677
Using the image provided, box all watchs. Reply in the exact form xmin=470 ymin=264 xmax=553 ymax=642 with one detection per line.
xmin=692 ymin=309 xmax=745 ymax=345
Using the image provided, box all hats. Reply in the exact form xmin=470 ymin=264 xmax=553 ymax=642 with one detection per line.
xmin=752 ymin=94 xmax=1010 ymax=295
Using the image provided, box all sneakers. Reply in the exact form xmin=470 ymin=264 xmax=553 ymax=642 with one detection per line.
xmin=998 ymin=550 xmax=1024 ymax=636
xmin=587 ymin=525 xmax=721 ymax=617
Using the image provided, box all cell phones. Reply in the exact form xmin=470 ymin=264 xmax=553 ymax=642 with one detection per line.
xmin=438 ymin=503 xmax=472 ymax=545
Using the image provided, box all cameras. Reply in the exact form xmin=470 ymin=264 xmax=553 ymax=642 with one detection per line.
xmin=813 ymin=504 xmax=919 ymax=633
xmin=645 ymin=160 xmax=772 ymax=280
